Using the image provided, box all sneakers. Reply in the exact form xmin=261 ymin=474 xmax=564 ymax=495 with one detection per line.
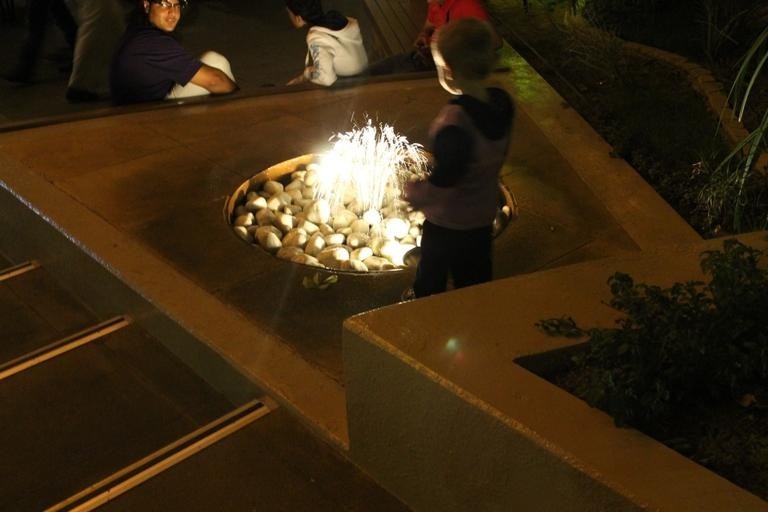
xmin=400 ymin=285 xmax=416 ymax=303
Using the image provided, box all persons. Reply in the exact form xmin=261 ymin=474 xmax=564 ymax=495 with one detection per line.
xmin=62 ymin=0 xmax=130 ymax=105
xmin=374 ymin=1 xmax=491 ymax=76
xmin=107 ymin=1 xmax=238 ymax=106
xmin=403 ymin=16 xmax=514 ymax=298
xmin=274 ymin=1 xmax=369 ymax=88
xmin=4 ymin=0 xmax=81 ymax=85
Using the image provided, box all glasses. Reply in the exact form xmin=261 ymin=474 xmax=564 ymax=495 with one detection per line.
xmin=152 ymin=0 xmax=184 ymax=9
